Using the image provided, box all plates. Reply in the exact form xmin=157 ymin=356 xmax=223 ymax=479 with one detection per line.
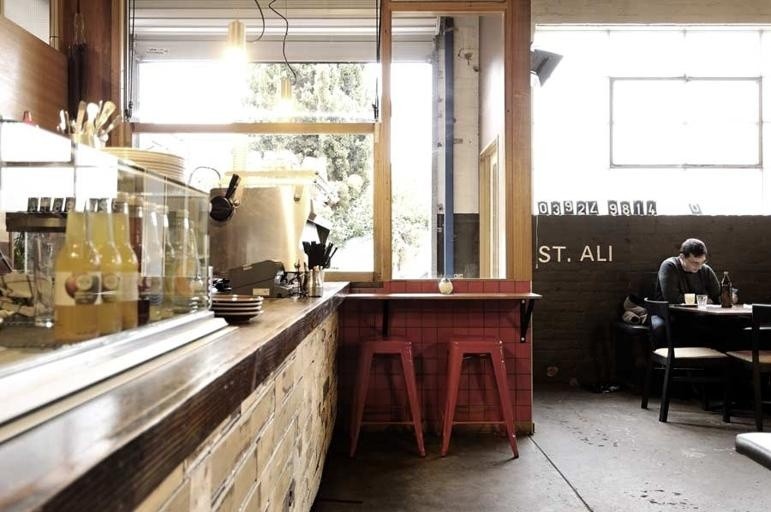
xmin=208 ymin=294 xmax=264 ymax=322
xmin=100 ymin=147 xmax=187 ymax=183
xmin=679 ymin=303 xmax=698 ymax=307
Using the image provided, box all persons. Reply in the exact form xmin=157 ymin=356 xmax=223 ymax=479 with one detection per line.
xmin=651 ymin=238 xmax=734 ymax=347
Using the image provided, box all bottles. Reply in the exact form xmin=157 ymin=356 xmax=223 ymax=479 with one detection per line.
xmin=439 ymin=278 xmax=454 ymax=294
xmin=721 ymin=271 xmax=732 ymax=308
xmin=5 ymin=197 xmax=203 ymax=348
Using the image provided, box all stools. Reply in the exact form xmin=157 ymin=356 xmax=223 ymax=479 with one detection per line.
xmin=438 ymin=336 xmax=521 ymax=458
xmin=347 ymin=334 xmax=427 ymax=460
xmin=612 ymin=318 xmax=656 ymax=396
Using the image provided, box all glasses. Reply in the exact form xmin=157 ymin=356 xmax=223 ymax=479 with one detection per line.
xmin=685 ymin=257 xmax=708 ymax=266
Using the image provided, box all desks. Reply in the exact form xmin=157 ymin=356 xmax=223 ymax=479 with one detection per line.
xmin=334 ymin=292 xmax=542 ymax=343
xmin=669 ymin=302 xmax=753 ymax=405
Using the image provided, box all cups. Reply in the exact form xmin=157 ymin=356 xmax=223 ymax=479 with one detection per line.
xmin=684 ymin=293 xmax=695 ymax=305
xmin=696 ymin=295 xmax=708 ymax=311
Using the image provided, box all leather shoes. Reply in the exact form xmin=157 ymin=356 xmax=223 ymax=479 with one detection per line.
xmin=704 ymin=400 xmax=737 ymax=411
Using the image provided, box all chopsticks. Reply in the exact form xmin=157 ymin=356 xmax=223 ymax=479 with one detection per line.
xmin=301 ymin=240 xmax=338 ymax=270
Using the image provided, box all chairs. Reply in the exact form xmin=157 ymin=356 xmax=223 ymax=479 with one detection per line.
xmin=721 ymin=305 xmax=771 ymax=431
xmin=641 ymin=296 xmax=734 ymax=424
